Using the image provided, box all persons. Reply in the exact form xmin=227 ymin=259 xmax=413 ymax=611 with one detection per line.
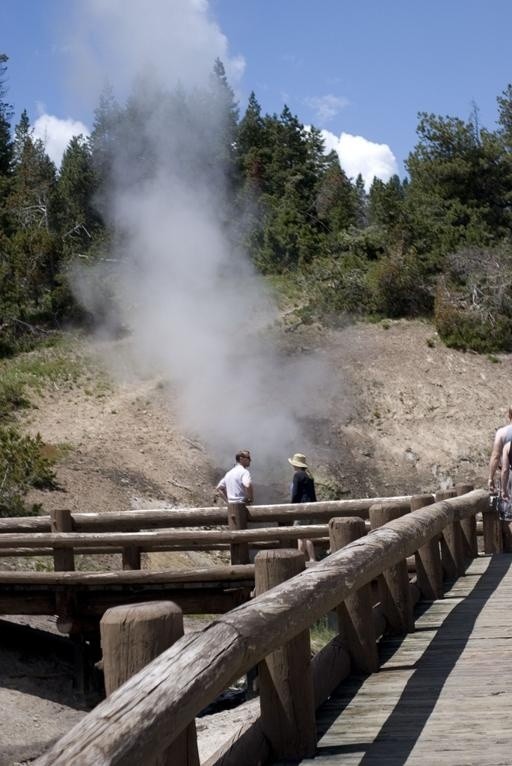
xmin=287 ymin=451 xmax=317 ymax=562
xmin=500 ymin=438 xmax=512 ymax=535
xmin=214 ymin=448 xmax=255 ymax=505
xmin=485 ymin=402 xmax=512 ymax=525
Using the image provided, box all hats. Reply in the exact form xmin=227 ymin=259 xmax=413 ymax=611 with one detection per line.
xmin=287 ymin=453 xmax=309 ymax=468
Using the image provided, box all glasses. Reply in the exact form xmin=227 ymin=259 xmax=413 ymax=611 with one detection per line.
xmin=244 ymin=456 xmax=250 ymax=459
